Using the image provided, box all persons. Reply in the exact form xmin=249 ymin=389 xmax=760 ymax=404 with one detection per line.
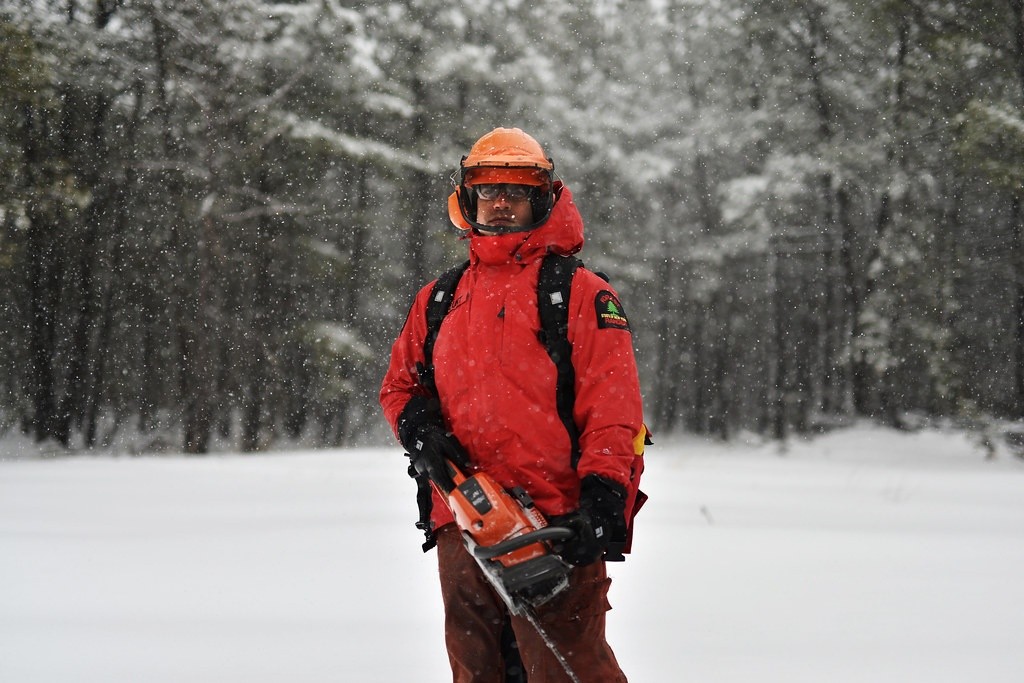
xmin=379 ymin=126 xmax=653 ymax=683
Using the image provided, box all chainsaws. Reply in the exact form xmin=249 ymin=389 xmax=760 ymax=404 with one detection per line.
xmin=415 ymin=453 xmax=590 ymax=683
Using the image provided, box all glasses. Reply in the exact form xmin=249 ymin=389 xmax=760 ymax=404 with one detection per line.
xmin=475 ymin=184 xmax=535 ymax=201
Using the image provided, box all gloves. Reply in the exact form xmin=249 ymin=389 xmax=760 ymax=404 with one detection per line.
xmin=562 ymin=474 xmax=628 ymax=566
xmin=397 ymin=399 xmax=468 ymax=493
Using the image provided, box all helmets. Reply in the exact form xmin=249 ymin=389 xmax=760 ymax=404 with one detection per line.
xmin=462 ymin=126 xmax=554 ymax=189
